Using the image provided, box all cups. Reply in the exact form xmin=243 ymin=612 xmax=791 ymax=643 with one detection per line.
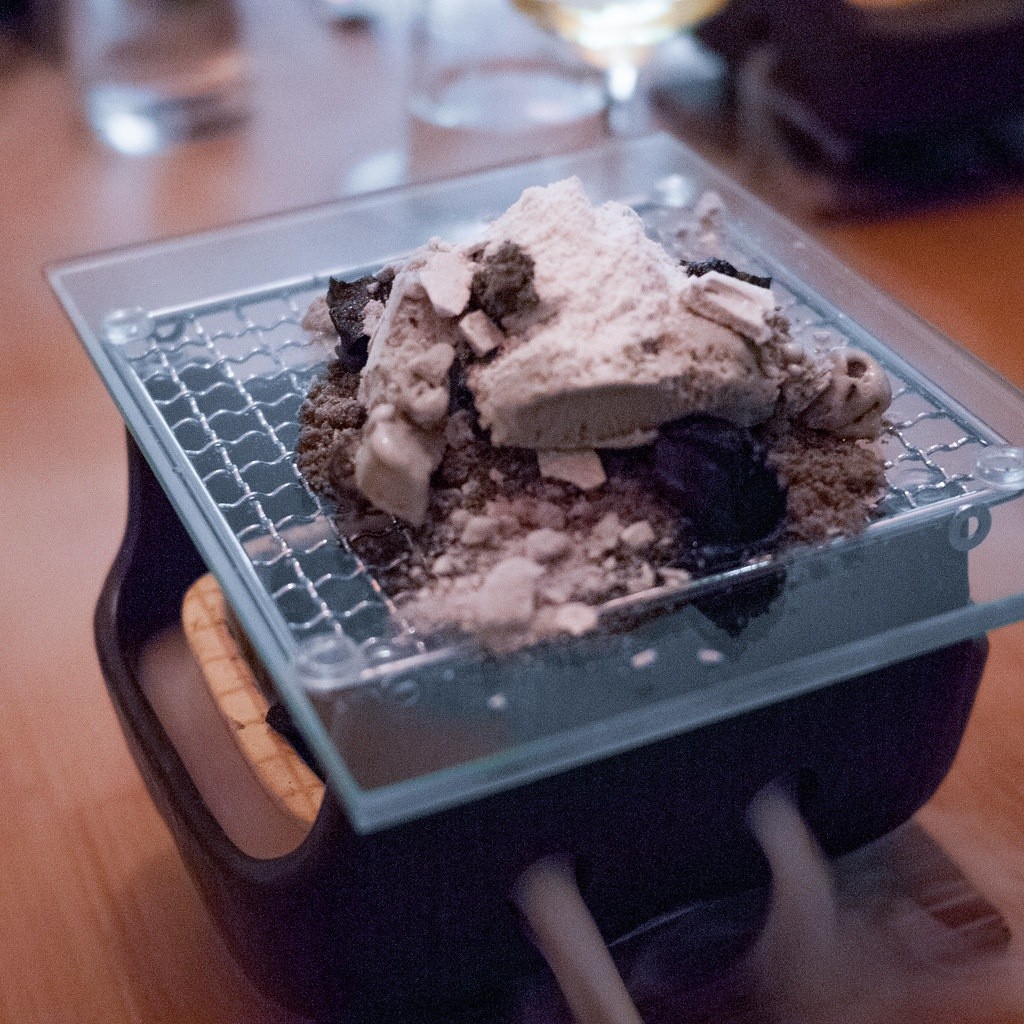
xmin=406 ymin=0 xmax=607 ymax=126
xmin=54 ymin=0 xmax=249 ymax=146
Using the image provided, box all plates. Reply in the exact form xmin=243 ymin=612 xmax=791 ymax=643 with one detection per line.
xmin=41 ymin=125 xmax=1024 ymax=834
